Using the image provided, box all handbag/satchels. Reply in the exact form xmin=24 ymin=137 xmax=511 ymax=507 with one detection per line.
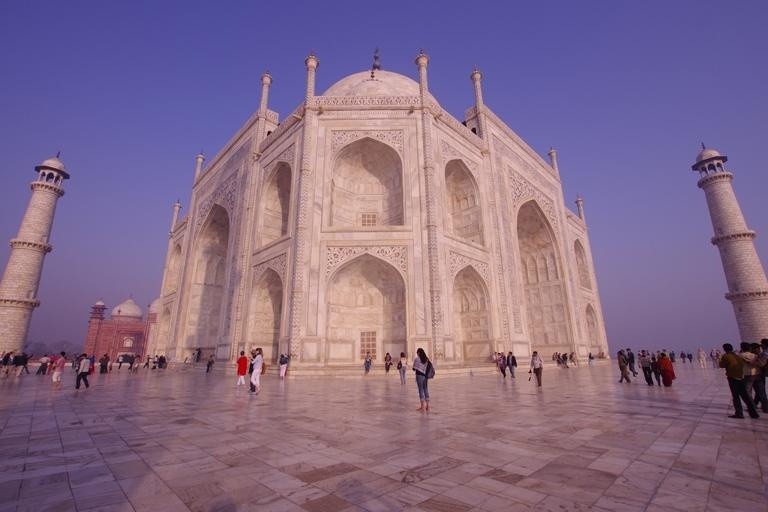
xmin=397 ymin=360 xmax=402 ymax=369
xmin=260 ymin=362 xmax=266 ymax=375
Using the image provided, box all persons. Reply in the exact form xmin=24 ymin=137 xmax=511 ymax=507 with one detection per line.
xmin=363 ymin=350 xmax=371 ymax=372
xmin=492 ymin=338 xmax=767 ymax=419
xmin=384 ymin=353 xmax=391 ymax=373
xmin=397 ymin=351 xmax=406 ymax=385
xmin=0 ymin=347 xmax=291 ymax=397
xmin=411 ymin=348 xmax=431 ymax=412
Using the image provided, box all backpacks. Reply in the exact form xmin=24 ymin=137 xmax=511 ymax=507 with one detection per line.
xmin=425 ymin=360 xmax=435 ymax=379
